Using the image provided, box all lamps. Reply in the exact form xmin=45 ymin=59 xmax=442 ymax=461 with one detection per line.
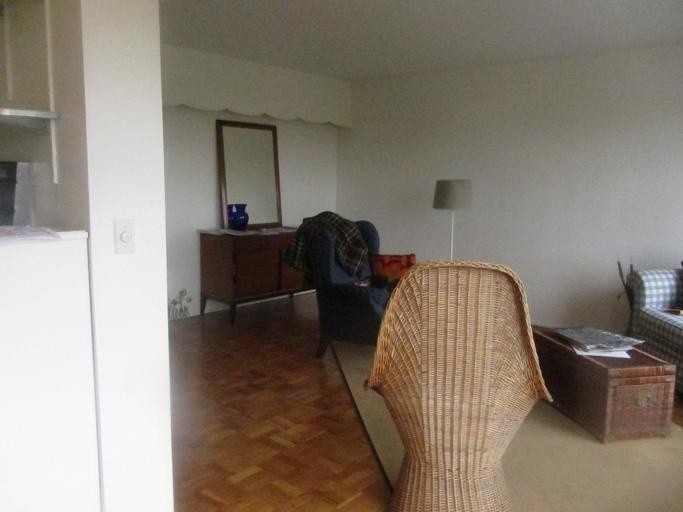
xmin=433 ymin=179 xmax=476 ymax=261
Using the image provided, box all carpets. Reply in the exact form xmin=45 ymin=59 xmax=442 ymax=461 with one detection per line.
xmin=330 ymin=336 xmax=683 ymax=511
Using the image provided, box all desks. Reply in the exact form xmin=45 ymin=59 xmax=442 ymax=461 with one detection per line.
xmin=532 ymin=326 xmax=676 ymax=446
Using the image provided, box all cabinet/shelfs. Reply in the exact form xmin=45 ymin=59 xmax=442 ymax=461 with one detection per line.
xmin=198 ymin=227 xmax=317 ymax=325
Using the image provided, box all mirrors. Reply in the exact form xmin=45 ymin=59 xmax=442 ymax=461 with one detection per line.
xmin=215 ymin=118 xmax=284 ymax=230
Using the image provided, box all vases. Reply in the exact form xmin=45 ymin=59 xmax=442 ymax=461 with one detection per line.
xmin=233 ymin=203 xmax=249 ymax=231
xmin=227 ymin=204 xmax=236 ymax=224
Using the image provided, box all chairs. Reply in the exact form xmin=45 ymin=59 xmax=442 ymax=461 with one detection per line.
xmin=364 ymin=260 xmax=555 ymax=511
xmin=625 ymin=268 xmax=683 ymax=395
xmin=306 ymin=221 xmax=395 ymax=359
